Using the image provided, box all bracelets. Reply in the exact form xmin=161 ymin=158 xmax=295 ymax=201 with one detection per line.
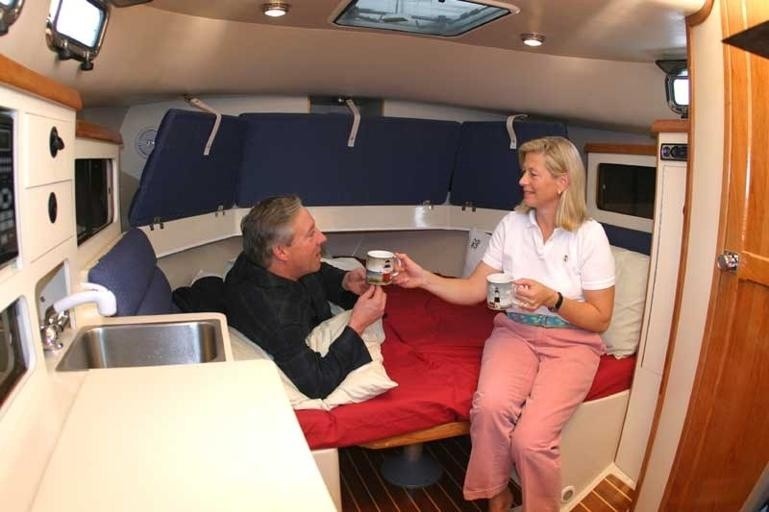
xmin=548 ymin=292 xmax=563 ymax=312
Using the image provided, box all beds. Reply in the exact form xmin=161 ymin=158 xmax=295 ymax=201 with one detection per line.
xmin=82 ymin=229 xmax=650 ymax=493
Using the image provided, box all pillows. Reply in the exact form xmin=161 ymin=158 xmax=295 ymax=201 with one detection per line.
xmin=211 ymin=315 xmax=399 ymax=412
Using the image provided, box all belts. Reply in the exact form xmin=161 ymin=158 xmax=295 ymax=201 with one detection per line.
xmin=506 ymin=312 xmax=569 ymax=328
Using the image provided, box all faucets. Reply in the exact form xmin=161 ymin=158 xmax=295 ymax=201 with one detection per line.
xmin=43 ymin=282 xmax=118 ymax=328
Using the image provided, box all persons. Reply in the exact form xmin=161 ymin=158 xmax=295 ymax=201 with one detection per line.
xmin=390 ymin=135 xmax=615 ymax=512
xmin=217 ymin=192 xmax=388 ymax=400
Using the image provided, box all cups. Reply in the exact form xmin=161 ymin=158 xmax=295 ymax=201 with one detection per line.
xmin=364 ymin=249 xmax=402 ymax=286
xmin=485 ymin=273 xmax=525 ymax=312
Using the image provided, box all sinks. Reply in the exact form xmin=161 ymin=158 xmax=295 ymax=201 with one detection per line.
xmin=55 ymin=319 xmax=227 ymax=373
